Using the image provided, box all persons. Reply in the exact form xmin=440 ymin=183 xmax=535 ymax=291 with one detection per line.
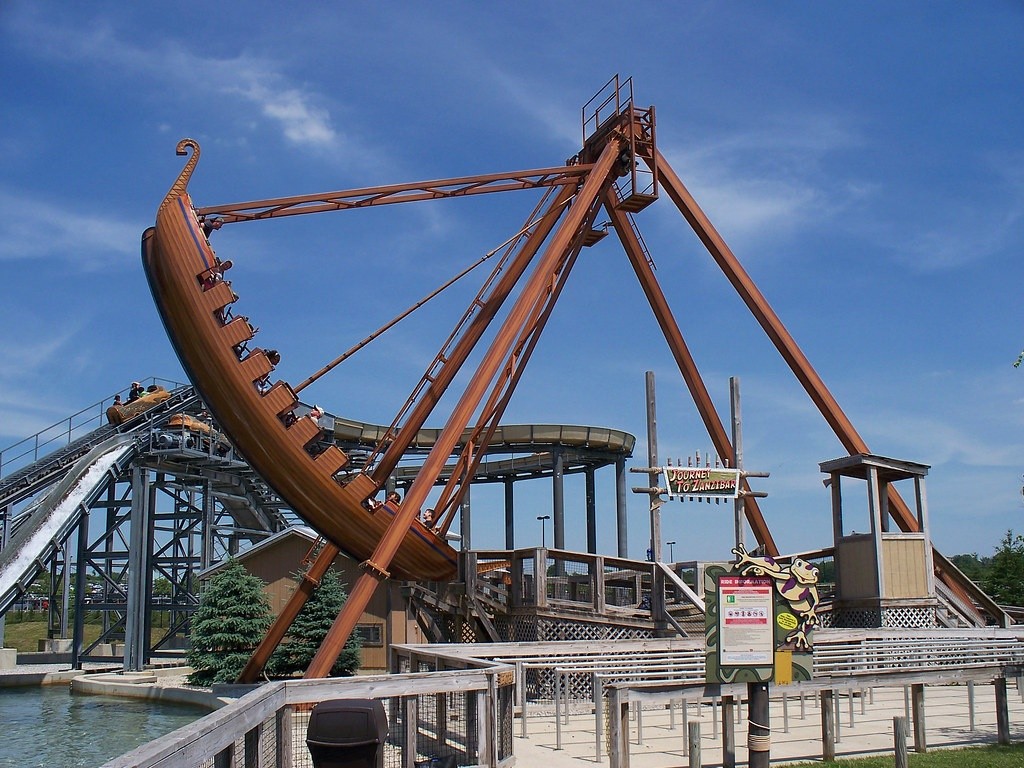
xmin=200 ymin=217 xmax=224 ymax=238
xmin=114 ymin=395 xmax=122 ymax=406
xmin=423 ymin=508 xmax=435 ymax=529
xmin=207 ymin=257 xmax=233 ymax=283
xmin=129 ymin=382 xmax=149 ymax=403
xmin=266 ymin=350 xmax=280 ymax=365
xmin=366 ymin=491 xmax=400 ymax=512
xmin=297 ymin=404 xmax=325 ymax=426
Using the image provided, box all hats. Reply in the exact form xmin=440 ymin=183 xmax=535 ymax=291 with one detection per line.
xmin=212 ymin=217 xmax=224 ymax=223
xmin=314 ymin=404 xmax=324 ymax=418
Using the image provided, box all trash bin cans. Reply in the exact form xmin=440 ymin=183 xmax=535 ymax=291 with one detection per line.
xmin=305 ymin=696 xmax=389 ymax=768
